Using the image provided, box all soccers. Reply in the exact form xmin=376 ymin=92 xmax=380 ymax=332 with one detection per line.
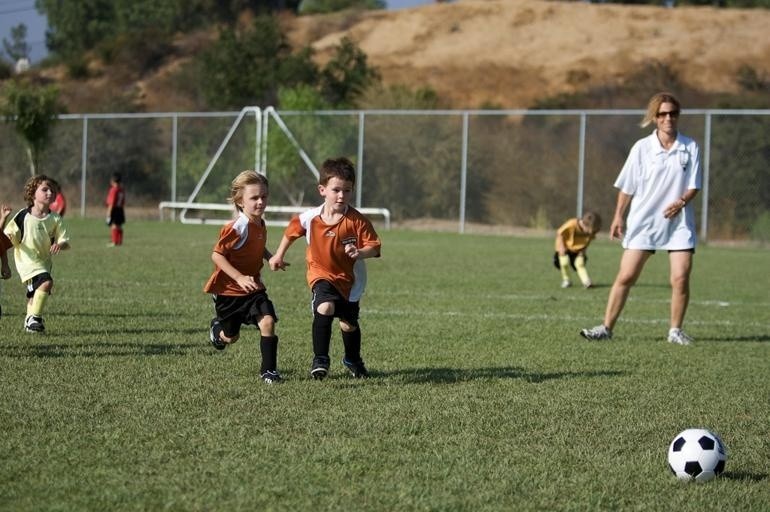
xmin=668 ymin=430 xmax=725 ymax=482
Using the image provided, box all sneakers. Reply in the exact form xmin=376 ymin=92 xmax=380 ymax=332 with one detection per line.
xmin=210 ymin=317 xmax=226 ymax=350
xmin=260 ymin=368 xmax=280 ymax=384
xmin=580 ymin=324 xmax=612 ymax=341
xmin=667 ymin=328 xmax=696 ymax=345
xmin=561 ymin=280 xmax=571 ymax=288
xmin=311 ymin=355 xmax=330 ymax=378
xmin=26 ymin=315 xmax=44 ymax=332
xmin=584 ymin=281 xmax=592 ymax=287
xmin=342 ymin=356 xmax=368 ymax=379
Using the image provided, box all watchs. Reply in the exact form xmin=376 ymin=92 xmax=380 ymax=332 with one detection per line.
xmin=681 ymin=198 xmax=686 ymax=208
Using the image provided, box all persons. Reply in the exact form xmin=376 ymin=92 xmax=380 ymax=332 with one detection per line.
xmin=553 ymin=210 xmax=604 ymax=289
xmin=268 ymin=156 xmax=384 ymax=379
xmin=48 ymin=185 xmax=67 ymax=219
xmin=0 ymin=173 xmax=72 ymax=334
xmin=201 ymin=168 xmax=292 ymax=386
xmin=579 ymin=92 xmax=703 ymax=347
xmin=103 ymin=172 xmax=126 ymax=249
xmin=0 ymin=203 xmax=13 ymax=281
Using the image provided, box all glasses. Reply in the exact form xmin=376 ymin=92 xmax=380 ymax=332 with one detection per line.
xmin=657 ymin=110 xmax=678 ymax=118
